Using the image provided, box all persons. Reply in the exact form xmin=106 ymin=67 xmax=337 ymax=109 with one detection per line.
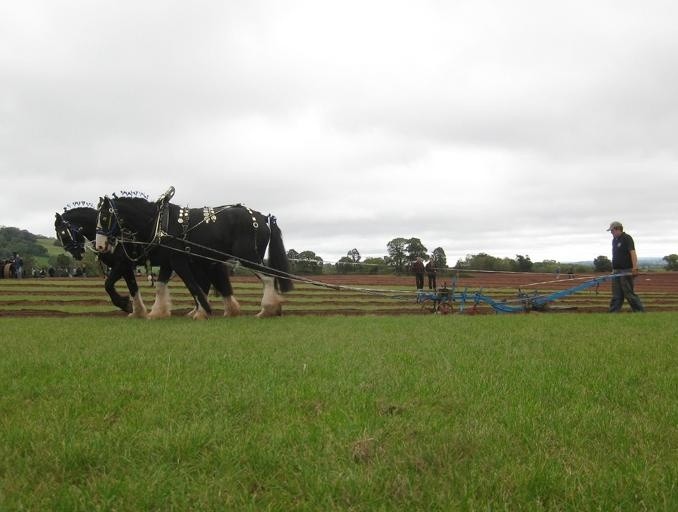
xmin=411 ymin=256 xmax=425 ymax=289
xmin=426 ymin=257 xmax=437 ymax=289
xmin=605 ymin=222 xmax=646 ymax=313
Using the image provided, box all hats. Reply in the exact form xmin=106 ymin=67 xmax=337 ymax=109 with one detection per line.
xmin=606 ymin=222 xmax=623 ymax=231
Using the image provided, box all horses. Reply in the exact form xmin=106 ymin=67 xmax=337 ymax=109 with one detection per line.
xmin=53 ymin=188 xmax=294 ymax=320
